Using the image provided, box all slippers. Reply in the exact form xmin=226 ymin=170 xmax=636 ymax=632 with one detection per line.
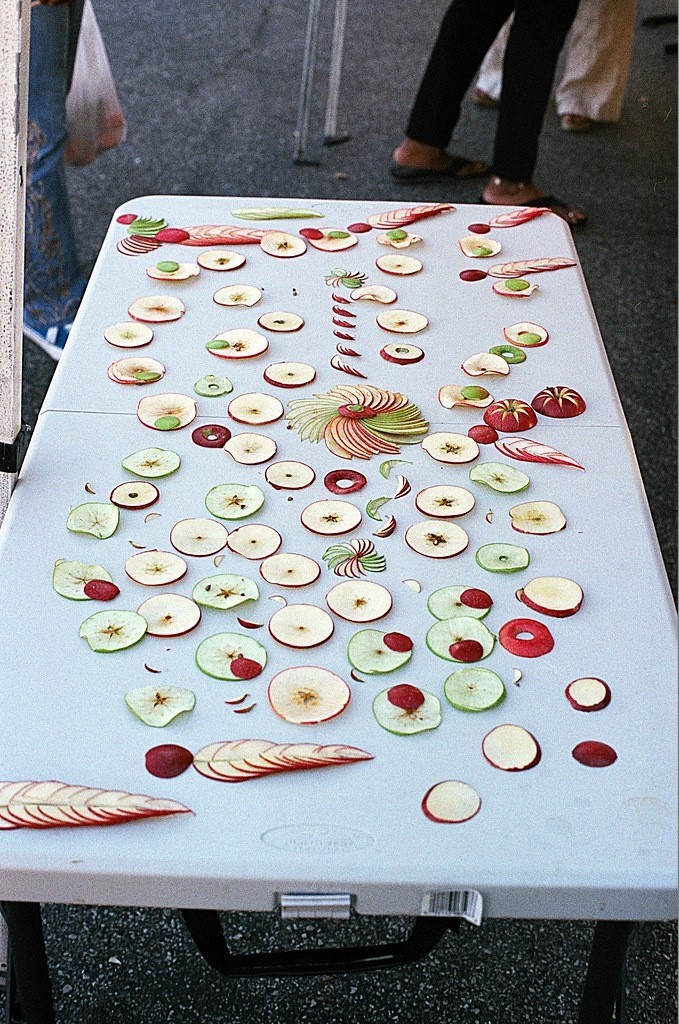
xmin=389 ymin=146 xmax=492 ymax=181
xmin=479 ymin=195 xmax=587 ymax=227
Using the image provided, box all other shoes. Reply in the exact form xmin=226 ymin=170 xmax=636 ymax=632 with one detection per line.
xmin=472 ymin=87 xmax=497 ymax=108
xmin=562 ymin=113 xmax=590 ymax=132
xmin=22 ymin=309 xmax=74 ymax=361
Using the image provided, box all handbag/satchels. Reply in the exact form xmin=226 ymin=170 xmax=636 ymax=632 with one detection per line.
xmin=64 ymin=0 xmax=126 ymax=166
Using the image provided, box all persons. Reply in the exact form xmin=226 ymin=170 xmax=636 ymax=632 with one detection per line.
xmin=389 ymin=1 xmax=642 ymax=230
xmin=23 ymin=1 xmax=81 ymax=364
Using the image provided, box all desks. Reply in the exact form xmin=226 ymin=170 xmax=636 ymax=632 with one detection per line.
xmin=0 ymin=196 xmax=679 ymax=1024
